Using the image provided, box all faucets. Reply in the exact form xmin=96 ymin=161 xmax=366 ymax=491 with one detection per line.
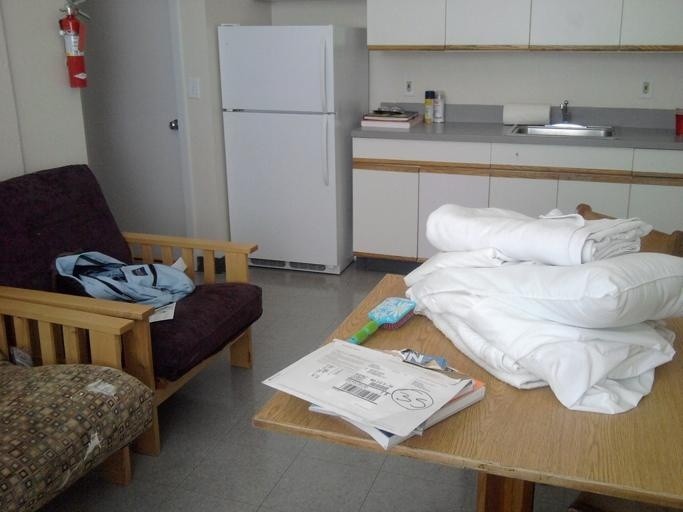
xmin=559 ymin=99 xmax=570 ymax=122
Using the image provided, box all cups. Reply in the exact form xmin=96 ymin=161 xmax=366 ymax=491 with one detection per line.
xmin=673 ymin=114 xmax=683 ymax=135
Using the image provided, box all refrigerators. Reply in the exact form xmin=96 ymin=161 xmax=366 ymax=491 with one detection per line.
xmin=216 ymin=19 xmax=371 ymax=276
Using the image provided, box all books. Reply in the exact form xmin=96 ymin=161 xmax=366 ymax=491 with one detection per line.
xmin=307 ymin=350 xmax=486 ymax=451
xmin=361 ymin=111 xmax=420 ymax=129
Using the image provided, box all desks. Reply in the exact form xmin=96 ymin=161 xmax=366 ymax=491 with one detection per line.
xmin=251 ymin=272 xmax=683 ymax=512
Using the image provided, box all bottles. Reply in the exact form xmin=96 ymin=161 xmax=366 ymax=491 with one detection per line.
xmin=423 ymin=90 xmax=435 ymax=123
xmin=434 ymin=89 xmax=445 ymax=124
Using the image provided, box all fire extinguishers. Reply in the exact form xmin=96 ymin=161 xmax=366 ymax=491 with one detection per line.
xmin=58 ymin=0 xmax=91 ymax=88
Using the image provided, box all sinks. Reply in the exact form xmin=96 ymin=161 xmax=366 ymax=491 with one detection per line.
xmin=508 ymin=123 xmax=619 ymax=140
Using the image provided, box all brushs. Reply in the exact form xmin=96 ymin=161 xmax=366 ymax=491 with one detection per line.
xmin=347 ymin=297 xmax=416 ymax=345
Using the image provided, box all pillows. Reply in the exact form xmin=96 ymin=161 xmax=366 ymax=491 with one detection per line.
xmin=409 ymin=251 xmax=683 ymax=328
